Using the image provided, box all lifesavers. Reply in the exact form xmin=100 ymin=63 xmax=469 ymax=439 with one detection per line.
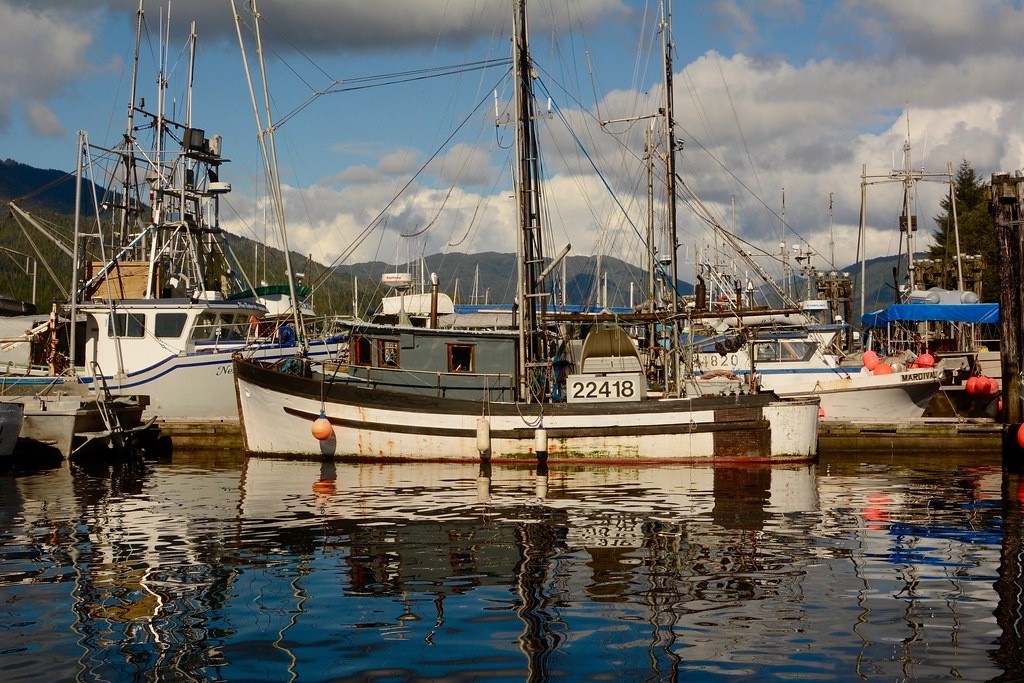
xmin=280 ymin=327 xmax=294 ymax=343
xmin=718 ymin=295 xmax=729 ymax=312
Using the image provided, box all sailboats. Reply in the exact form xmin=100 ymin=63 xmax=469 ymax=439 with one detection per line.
xmin=0 ymin=0 xmax=1006 ymax=465
